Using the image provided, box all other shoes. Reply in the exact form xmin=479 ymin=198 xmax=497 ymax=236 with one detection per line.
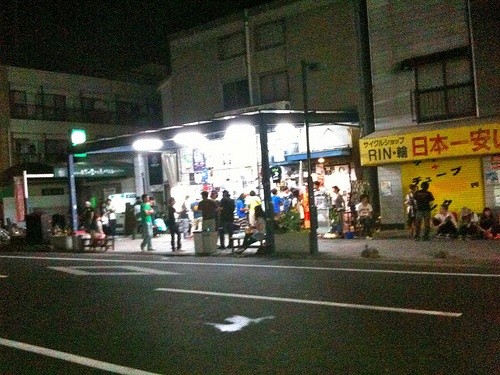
xmin=147 ymin=247 xmax=157 ymax=251
xmin=140 ymin=245 xmax=145 ymax=252
xmin=177 ymin=248 xmax=186 ymax=252
xmin=227 ymin=244 xmax=234 ymax=248
xmin=218 ymin=246 xmax=226 ymax=249
xmin=436 ymin=232 xmax=472 ymax=241
xmin=173 ymin=249 xmax=179 ymax=252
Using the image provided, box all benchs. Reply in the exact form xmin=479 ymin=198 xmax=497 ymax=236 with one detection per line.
xmin=82 ymin=236 xmax=114 ymax=252
xmin=229 ymin=236 xmax=266 ymax=253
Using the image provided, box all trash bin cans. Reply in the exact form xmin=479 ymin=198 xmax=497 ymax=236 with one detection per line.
xmin=23 ymin=210 xmax=51 ymax=246
xmin=192 ymin=231 xmax=220 ymax=254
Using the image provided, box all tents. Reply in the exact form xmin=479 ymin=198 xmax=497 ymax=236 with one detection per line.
xmin=66 ymin=108 xmax=363 ymax=255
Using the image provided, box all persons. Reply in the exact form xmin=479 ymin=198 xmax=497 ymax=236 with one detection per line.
xmin=140 ymin=194 xmax=155 ymax=251
xmin=411 ymin=182 xmax=435 ymax=241
xmin=82 ymin=178 xmax=500 ymax=251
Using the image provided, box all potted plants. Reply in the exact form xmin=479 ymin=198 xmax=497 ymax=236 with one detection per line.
xmin=274 ymin=205 xmax=311 ymax=255
